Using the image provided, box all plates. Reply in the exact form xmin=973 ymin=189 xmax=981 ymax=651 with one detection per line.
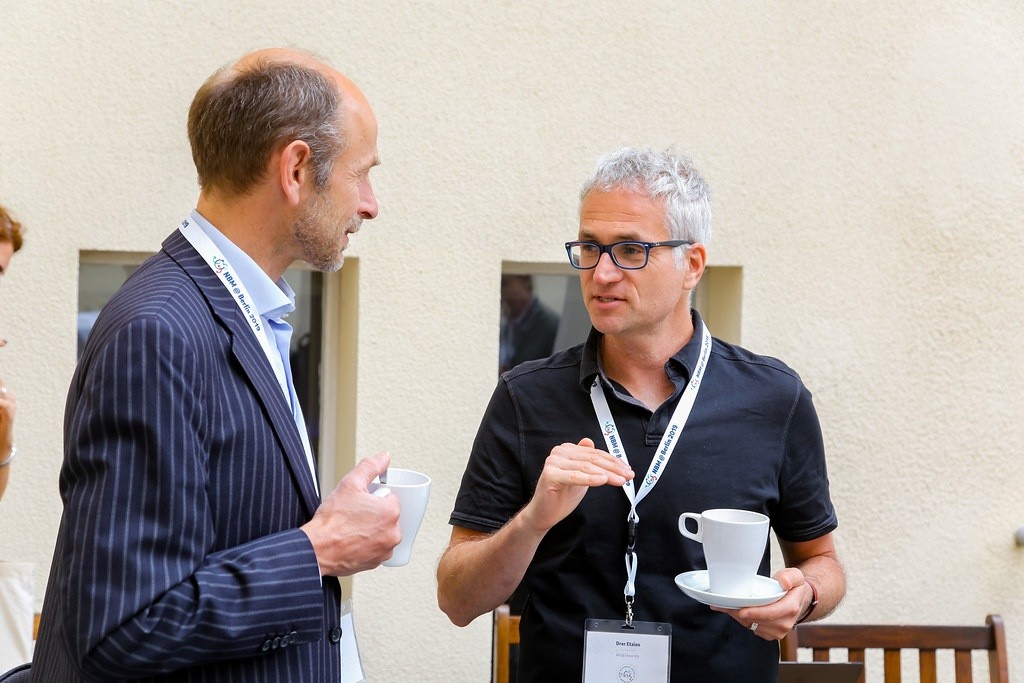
xmin=674 ymin=570 xmax=787 ymax=611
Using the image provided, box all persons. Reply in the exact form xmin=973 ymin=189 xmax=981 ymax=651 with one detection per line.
xmin=437 ymin=149 xmax=846 ymax=683
xmin=31 ymin=48 xmax=403 ymax=683
xmin=499 ymin=274 xmax=560 ymax=374
xmin=0 ymin=208 xmax=22 ymax=499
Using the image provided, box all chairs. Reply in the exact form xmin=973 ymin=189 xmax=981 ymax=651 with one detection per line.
xmin=781 ymin=614 xmax=1012 ymax=682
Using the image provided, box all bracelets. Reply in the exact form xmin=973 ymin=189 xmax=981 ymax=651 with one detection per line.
xmin=0 ymin=444 xmax=16 ymax=467
xmin=795 ymin=581 xmax=819 ymax=626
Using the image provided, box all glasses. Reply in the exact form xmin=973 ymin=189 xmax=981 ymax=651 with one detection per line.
xmin=565 ymin=240 xmax=694 ymax=270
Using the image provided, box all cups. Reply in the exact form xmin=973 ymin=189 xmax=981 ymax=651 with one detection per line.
xmin=679 ymin=509 xmax=769 ymax=597
xmin=368 ymin=468 xmax=431 ymax=568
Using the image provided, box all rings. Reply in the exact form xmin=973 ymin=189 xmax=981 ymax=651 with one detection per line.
xmin=2 ymin=388 xmax=7 ymax=394
xmin=749 ymin=623 xmax=758 ymax=631
